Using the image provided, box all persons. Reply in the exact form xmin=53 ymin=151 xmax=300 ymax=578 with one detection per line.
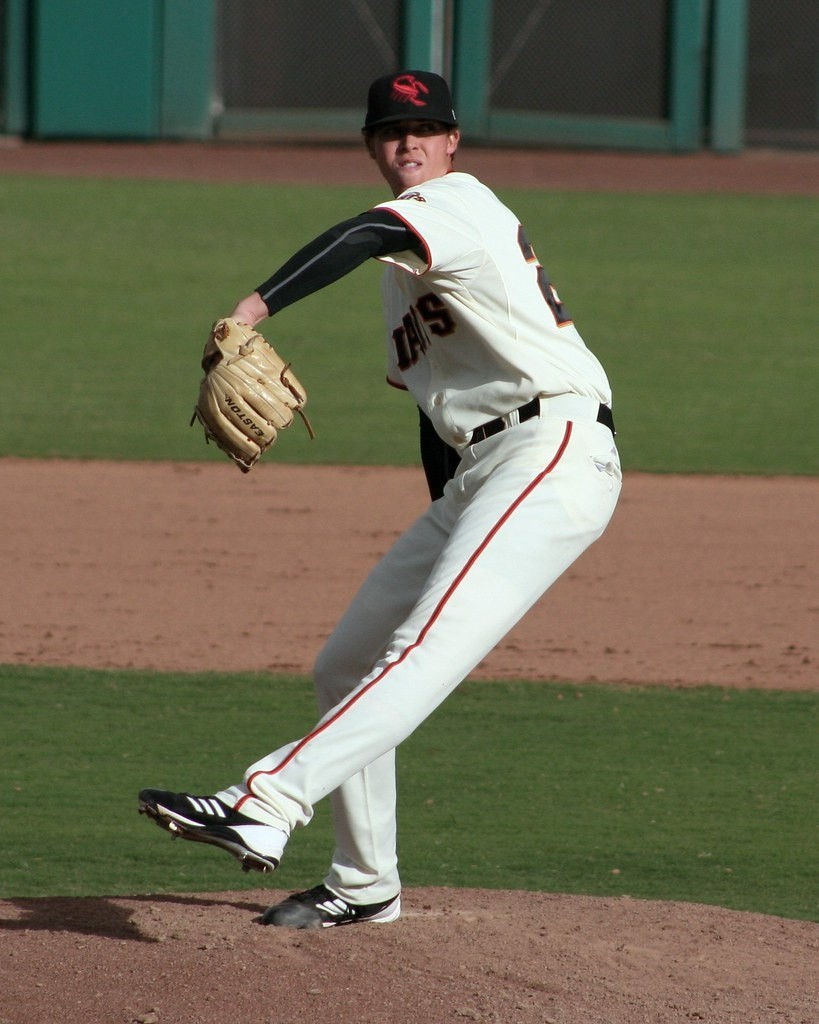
xmin=139 ymin=72 xmax=621 ymax=929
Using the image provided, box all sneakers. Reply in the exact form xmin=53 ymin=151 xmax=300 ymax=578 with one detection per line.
xmin=138 ymin=789 xmax=288 ymax=874
xmin=263 ymin=884 xmax=402 ymax=929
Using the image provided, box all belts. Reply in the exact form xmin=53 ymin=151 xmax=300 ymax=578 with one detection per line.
xmin=469 ymin=396 xmax=616 ymax=446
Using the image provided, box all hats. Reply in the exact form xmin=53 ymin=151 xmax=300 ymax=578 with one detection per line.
xmin=365 ymin=70 xmax=459 ymax=131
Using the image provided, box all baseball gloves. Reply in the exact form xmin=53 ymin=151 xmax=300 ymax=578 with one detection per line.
xmin=194 ymin=316 xmax=309 ymax=475
